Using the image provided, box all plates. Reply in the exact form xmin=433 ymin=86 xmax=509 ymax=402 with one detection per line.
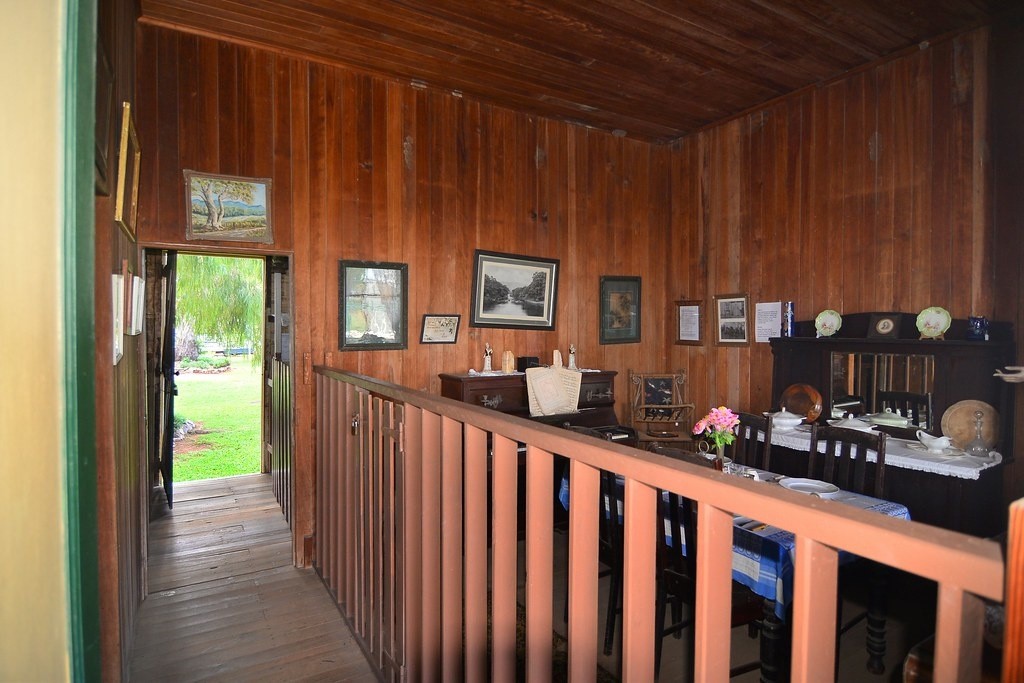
xmin=779 ymin=382 xmax=822 ymax=423
xmin=940 ymin=400 xmax=1000 ymax=452
xmin=703 ymin=453 xmax=732 ymax=465
xmin=916 ymin=306 xmax=952 ymax=337
xmin=815 ymin=309 xmax=842 ymax=337
xmin=778 ymin=478 xmax=840 ymax=496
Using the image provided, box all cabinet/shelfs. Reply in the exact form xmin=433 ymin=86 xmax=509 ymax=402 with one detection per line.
xmin=770 ymin=310 xmax=1015 ymax=460
xmin=628 ymin=368 xmax=697 ymax=451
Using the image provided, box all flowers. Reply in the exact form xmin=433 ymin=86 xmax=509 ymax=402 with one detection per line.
xmin=692 ymin=405 xmax=741 ymax=448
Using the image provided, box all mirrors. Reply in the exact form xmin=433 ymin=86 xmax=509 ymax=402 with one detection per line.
xmin=831 ymin=350 xmax=934 ymax=432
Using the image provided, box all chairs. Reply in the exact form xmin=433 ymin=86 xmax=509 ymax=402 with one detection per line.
xmin=878 ymin=388 xmax=933 ymax=431
xmin=807 ymin=425 xmax=885 ymax=500
xmin=902 ymin=497 xmax=1024 ymax=683
xmin=564 ymin=412 xmax=774 ymax=678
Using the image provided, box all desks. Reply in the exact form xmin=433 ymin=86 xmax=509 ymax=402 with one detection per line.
xmin=734 ymin=424 xmax=1006 ymax=539
xmin=555 ymin=463 xmax=912 ymax=683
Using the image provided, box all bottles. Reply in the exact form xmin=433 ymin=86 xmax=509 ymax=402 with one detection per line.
xmin=967 ymin=410 xmax=992 ymax=457
xmin=967 ymin=315 xmax=990 ymax=340
xmin=501 ymin=350 xmax=514 ymax=374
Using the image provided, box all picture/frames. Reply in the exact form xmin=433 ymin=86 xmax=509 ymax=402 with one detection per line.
xmin=114 ymin=101 xmax=141 ymax=244
xmin=599 ymin=275 xmax=642 ymax=345
xmin=183 ymin=168 xmax=275 ymax=245
xmin=113 ymin=259 xmax=146 ymax=365
xmin=336 ymin=259 xmax=408 ymax=351
xmin=712 ymin=292 xmax=752 ymax=348
xmin=420 ymin=314 xmax=462 ymax=344
xmin=867 ymin=313 xmax=902 ymax=339
xmin=468 ymin=249 xmax=562 ymax=331
xmin=641 ymin=374 xmax=675 ymax=421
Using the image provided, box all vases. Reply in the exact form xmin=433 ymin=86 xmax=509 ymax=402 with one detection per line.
xmin=717 ymin=444 xmax=724 ymax=461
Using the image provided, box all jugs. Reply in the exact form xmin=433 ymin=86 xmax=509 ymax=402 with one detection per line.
xmin=916 ymin=429 xmax=953 ymax=454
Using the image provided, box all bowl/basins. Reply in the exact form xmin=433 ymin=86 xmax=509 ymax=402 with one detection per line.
xmin=826 ymin=414 xmax=878 ymax=434
xmin=867 ymin=408 xmax=914 ymax=428
xmin=832 ymin=408 xmax=847 ymax=417
xmin=763 ymin=406 xmax=808 ymax=430
